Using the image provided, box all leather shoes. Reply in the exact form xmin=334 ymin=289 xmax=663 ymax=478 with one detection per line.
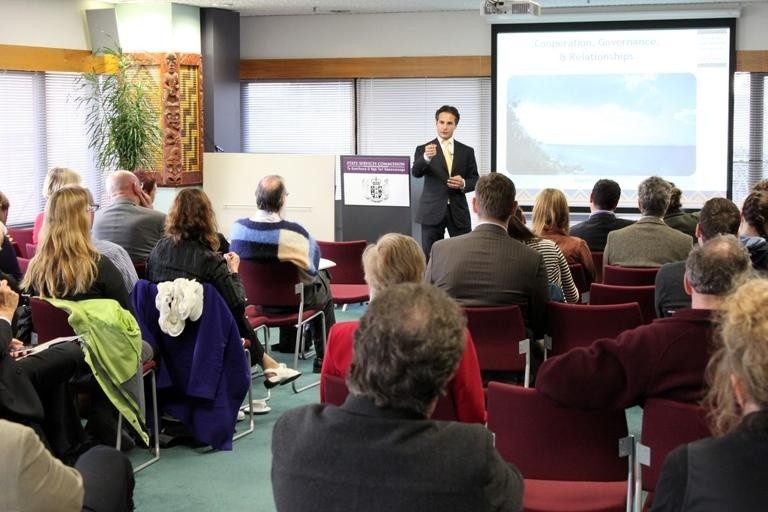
xmin=78 ymin=398 xmax=133 ymax=451
xmin=312 ymin=357 xmax=323 ymax=373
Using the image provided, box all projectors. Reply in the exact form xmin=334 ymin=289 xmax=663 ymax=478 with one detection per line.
xmin=480 ymin=0 xmax=541 ymax=18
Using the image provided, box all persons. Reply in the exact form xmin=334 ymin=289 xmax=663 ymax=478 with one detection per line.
xmin=426 ymin=172 xmax=768 ymax=510
xmin=1 ymin=418 xmax=135 ymax=511
xmin=270 ymin=282 xmax=524 ymax=511
xmin=320 ymin=233 xmax=488 ymax=424
xmin=409 ymin=105 xmax=479 ymax=261
xmin=0 ymin=168 xmax=336 ymax=465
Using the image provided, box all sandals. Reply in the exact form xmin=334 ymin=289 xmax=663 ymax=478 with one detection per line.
xmin=264 ymin=362 xmax=302 ymax=389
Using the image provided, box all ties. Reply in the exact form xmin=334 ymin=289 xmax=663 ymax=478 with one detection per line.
xmin=443 ymin=140 xmax=452 ymax=177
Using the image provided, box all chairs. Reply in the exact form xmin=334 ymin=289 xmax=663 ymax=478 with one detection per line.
xmin=241 ymin=257 xmax=335 ymax=412
xmin=310 ymin=229 xmax=741 ymax=512
xmin=1 ymin=229 xmax=254 ymax=474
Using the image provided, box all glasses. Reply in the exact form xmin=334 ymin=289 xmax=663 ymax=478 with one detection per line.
xmin=91 ymin=204 xmax=99 ymax=211
